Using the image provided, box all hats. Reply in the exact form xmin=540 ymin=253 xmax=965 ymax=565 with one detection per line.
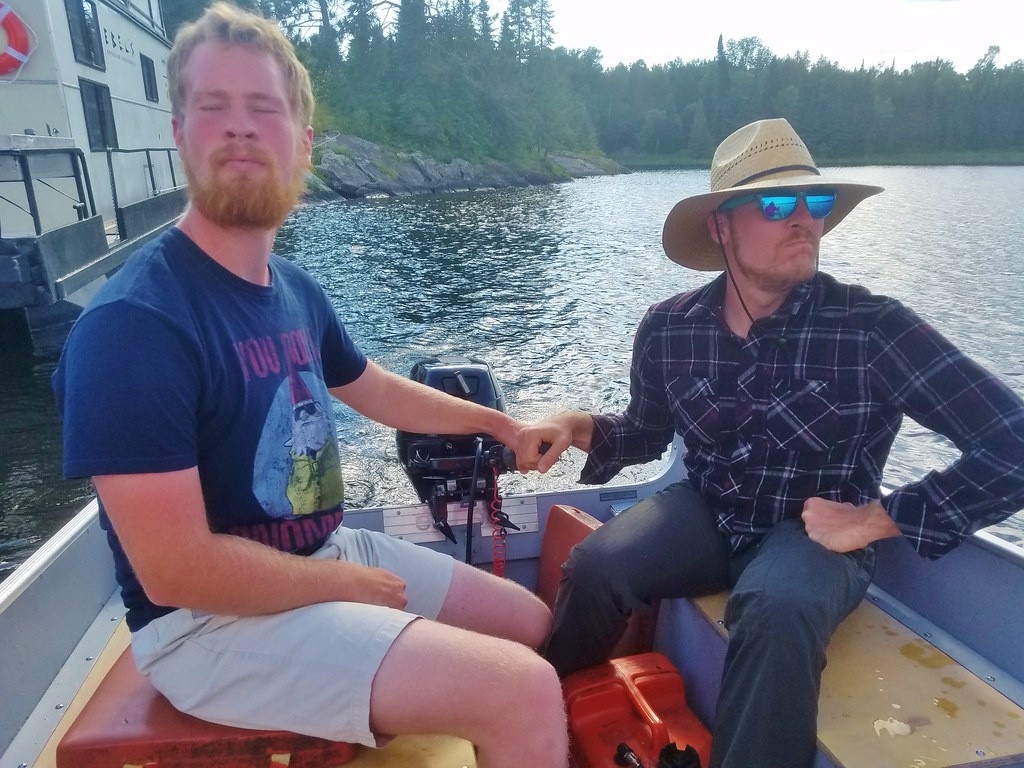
xmin=662 ymin=117 xmax=886 ymax=272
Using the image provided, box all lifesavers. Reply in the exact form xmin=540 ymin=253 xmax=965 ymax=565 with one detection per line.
xmin=0 ymin=2 xmax=30 ymax=76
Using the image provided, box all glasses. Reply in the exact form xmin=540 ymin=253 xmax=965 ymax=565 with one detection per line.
xmin=717 ymin=188 xmax=839 ymax=221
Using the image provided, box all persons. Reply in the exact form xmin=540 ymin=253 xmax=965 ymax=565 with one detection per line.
xmin=516 ymin=117 xmax=1024 ymax=768
xmin=59 ymin=2 xmax=569 ymax=768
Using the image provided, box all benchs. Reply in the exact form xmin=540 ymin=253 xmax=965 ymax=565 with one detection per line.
xmin=614 ymin=493 xmax=1024 ymax=768
xmin=39 ymin=545 xmax=475 ymax=768
